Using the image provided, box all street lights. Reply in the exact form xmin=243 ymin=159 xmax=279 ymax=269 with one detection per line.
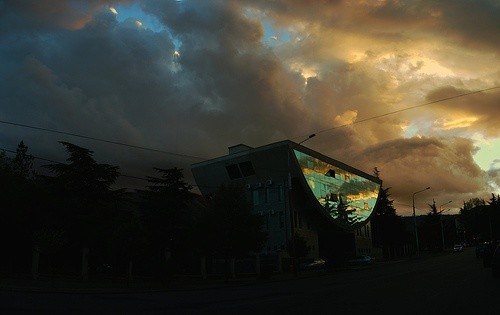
xmin=440 ymin=201 xmax=452 ymax=252
xmin=287 ymin=131 xmax=318 ymax=272
xmin=412 ymin=187 xmax=431 ymax=257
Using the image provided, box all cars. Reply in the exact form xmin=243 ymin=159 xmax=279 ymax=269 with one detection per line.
xmin=452 ymin=242 xmax=464 ymax=253
